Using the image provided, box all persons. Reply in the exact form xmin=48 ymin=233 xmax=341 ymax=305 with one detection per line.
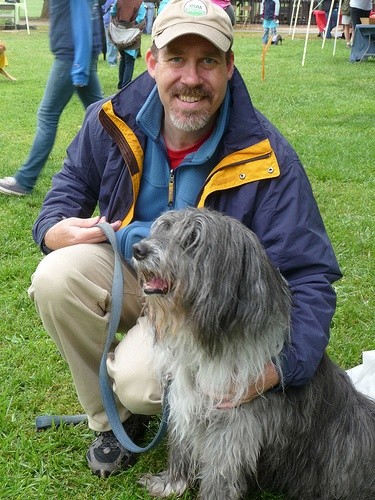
xmin=28 ymin=0 xmax=344 ymax=476
xmin=316 ymin=0 xmax=372 ymax=48
xmin=260 ymin=0 xmax=280 ymax=46
xmin=0 ymin=0 xmax=107 ymax=197
xmin=96 ymin=0 xmax=236 ymax=92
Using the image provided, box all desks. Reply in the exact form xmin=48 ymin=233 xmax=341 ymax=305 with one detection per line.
xmin=350 ymin=23 xmax=375 ymax=62
xmin=0 ymin=2 xmax=18 ymax=30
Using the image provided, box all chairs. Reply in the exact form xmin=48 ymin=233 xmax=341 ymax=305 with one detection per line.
xmin=234 ymin=6 xmax=252 ymax=31
xmin=0 ymin=4 xmax=17 ymax=32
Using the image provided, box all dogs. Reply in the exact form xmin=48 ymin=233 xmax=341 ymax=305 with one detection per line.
xmin=0 ymin=41 xmax=18 ymax=82
xmin=270 ymin=34 xmax=283 ymax=45
xmin=131 ymin=207 xmax=375 ymax=500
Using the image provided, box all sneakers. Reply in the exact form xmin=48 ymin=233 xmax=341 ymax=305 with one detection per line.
xmin=84 ymin=411 xmax=148 ymax=478
xmin=0 ymin=177 xmax=31 ymax=196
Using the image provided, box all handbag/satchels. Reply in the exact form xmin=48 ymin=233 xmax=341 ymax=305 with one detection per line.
xmin=108 ymin=17 xmax=147 ymax=48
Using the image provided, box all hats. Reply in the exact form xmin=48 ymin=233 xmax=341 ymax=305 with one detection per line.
xmin=152 ymin=0 xmax=234 ymax=52
